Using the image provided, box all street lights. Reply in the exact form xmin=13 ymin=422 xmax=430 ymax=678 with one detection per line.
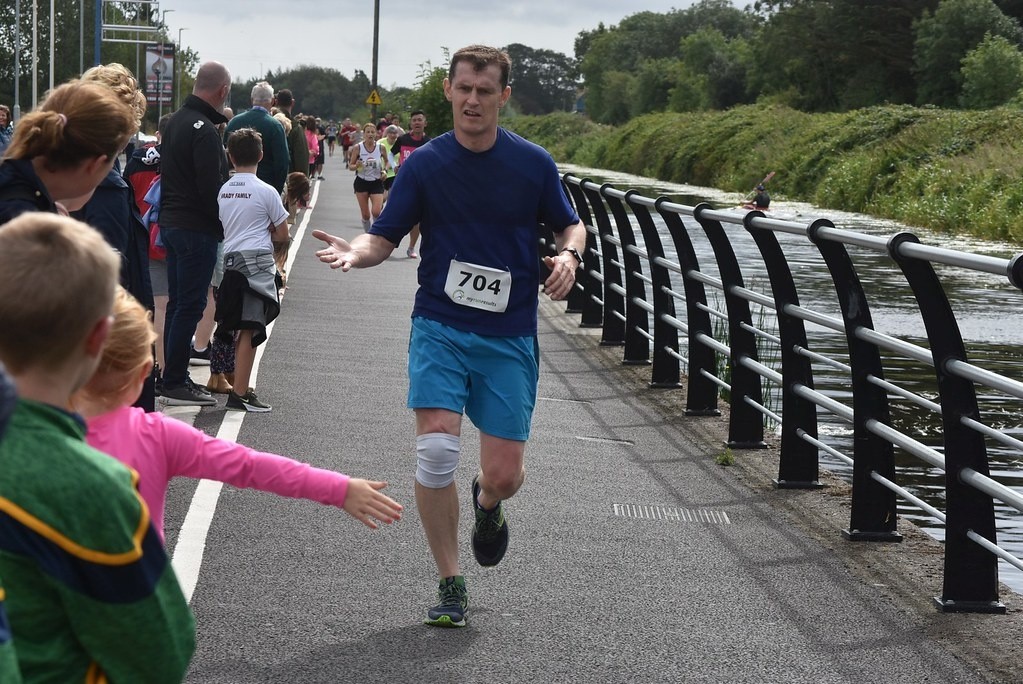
xmin=176 ymin=27 xmax=191 ymax=109
xmin=158 ymin=9 xmax=177 ymax=115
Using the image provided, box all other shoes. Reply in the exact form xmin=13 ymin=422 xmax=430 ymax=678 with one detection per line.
xmin=318 ymin=176 xmax=325 ymax=181
xmin=311 ymin=176 xmax=315 ymax=181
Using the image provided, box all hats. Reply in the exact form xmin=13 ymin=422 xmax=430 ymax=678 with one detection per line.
xmin=274 ymin=89 xmax=292 ymax=101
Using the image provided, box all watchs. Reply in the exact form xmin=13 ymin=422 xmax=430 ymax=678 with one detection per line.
xmin=561 ymin=247 xmax=583 ymax=264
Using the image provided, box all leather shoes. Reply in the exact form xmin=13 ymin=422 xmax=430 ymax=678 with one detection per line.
xmin=207 ymin=373 xmax=233 ymax=393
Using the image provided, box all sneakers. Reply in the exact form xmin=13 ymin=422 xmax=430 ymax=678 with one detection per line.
xmin=225 ymin=389 xmax=272 ymax=412
xmin=470 ymin=472 xmax=509 ymax=566
xmin=407 ymin=249 xmax=417 ymax=258
xmin=189 ymin=339 xmax=214 ymax=365
xmin=154 ymin=367 xmax=218 ymax=406
xmin=424 ymin=575 xmax=469 ymax=626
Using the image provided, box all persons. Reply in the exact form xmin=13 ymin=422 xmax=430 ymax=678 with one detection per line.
xmin=750 ymin=185 xmax=770 ymax=208
xmin=67 ymin=286 xmax=402 ymax=547
xmin=327 ymin=111 xmax=406 ymax=233
xmin=0 ymin=58 xmax=325 ymax=413
xmin=0 ymin=212 xmax=195 ymax=684
xmin=387 ymin=110 xmax=432 ymax=257
xmin=313 ymin=46 xmax=587 ymax=626
